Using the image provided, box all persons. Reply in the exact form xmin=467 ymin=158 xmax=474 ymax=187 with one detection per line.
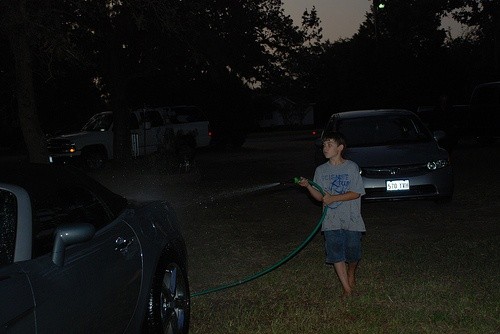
xmin=297 ymin=134 xmax=366 ymax=303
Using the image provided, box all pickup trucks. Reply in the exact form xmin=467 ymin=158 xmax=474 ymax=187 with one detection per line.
xmin=44 ymin=106 xmax=210 ymax=172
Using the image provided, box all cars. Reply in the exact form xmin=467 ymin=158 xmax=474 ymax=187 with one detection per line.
xmin=0 ymin=164 xmax=194 ymax=334
xmin=312 ymin=108 xmax=453 ymax=208
